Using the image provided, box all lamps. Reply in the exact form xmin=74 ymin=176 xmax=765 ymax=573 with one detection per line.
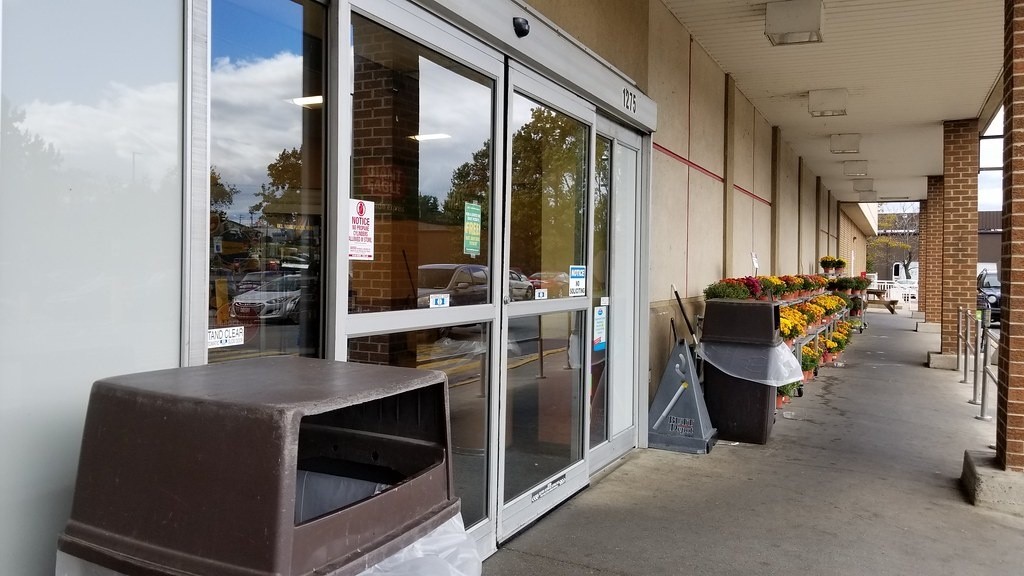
xmin=766 ymin=1 xmax=878 ymax=203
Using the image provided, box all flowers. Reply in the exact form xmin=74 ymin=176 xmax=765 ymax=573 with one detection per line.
xmin=819 ymin=255 xmax=836 ymax=269
xmin=704 ymin=273 xmax=871 ymax=396
xmin=835 ymin=258 xmax=847 ymax=268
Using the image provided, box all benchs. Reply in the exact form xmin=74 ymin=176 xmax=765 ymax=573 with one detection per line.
xmin=866 ymin=300 xmax=898 ymax=314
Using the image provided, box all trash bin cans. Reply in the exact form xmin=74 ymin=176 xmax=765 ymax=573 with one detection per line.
xmin=52 ymin=355 xmax=461 ymax=576
xmin=698 ymin=299 xmax=777 ymax=446
xmin=572 ymin=298 xmax=608 ymax=450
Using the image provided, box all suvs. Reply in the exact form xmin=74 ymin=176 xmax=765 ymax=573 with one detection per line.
xmin=976 ymin=268 xmax=1002 ymax=318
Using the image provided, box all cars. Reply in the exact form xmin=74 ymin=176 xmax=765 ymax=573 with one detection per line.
xmin=208 ymin=252 xmax=308 ymax=325
xmin=893 ymin=279 xmax=917 ymax=299
xmin=417 ymin=263 xmax=571 ymax=341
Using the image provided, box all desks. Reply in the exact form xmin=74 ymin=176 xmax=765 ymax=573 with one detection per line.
xmin=865 ymin=289 xmax=897 ymax=315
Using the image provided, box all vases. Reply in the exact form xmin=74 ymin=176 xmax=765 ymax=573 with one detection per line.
xmin=825 ymin=268 xmax=833 ymax=274
xmin=744 ymin=289 xmax=866 ymax=409
xmin=835 ymin=267 xmax=844 ymax=274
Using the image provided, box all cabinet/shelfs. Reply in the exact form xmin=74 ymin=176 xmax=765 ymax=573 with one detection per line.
xmin=776 ymin=291 xmax=867 ymax=406
